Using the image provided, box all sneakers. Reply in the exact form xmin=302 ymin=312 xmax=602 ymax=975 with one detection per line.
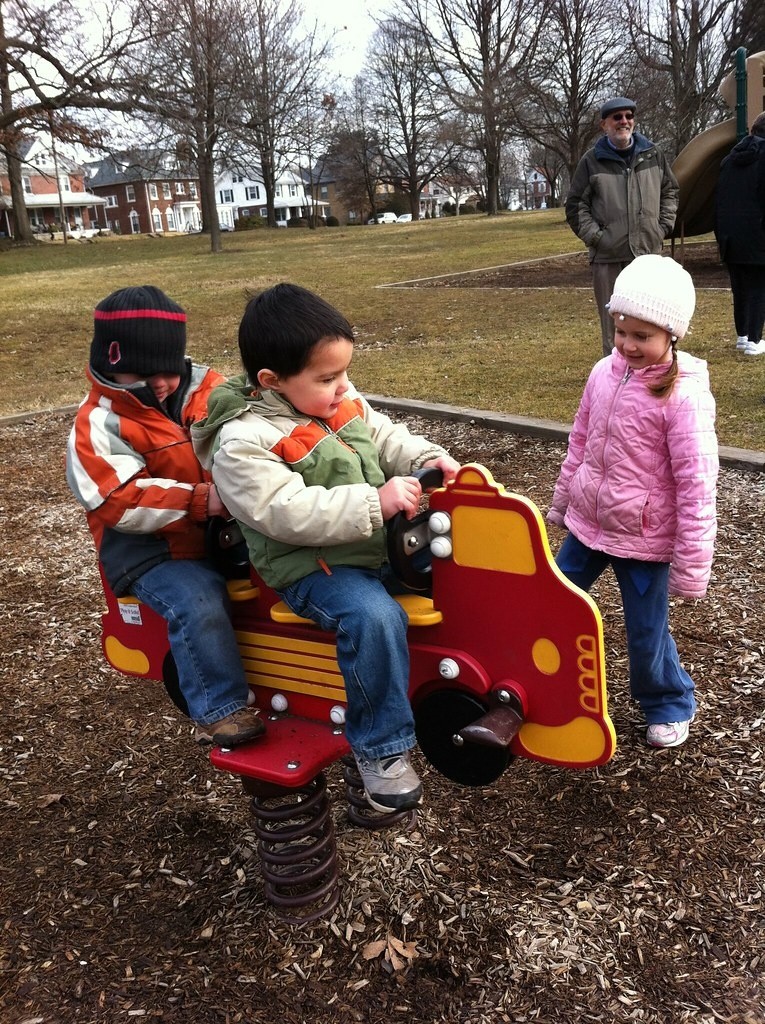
xmin=194 ymin=711 xmax=266 ymax=747
xmin=736 ymin=336 xmax=749 ymax=349
xmin=744 ymin=339 xmax=765 ymax=355
xmin=647 ymin=713 xmax=695 ymax=747
xmin=352 ymin=747 xmax=424 ymax=813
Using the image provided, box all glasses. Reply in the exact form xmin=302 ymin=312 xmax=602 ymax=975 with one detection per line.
xmin=613 ymin=113 xmax=635 ymax=121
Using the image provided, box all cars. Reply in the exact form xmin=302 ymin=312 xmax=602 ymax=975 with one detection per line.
xmin=396 ymin=214 xmax=420 ymax=222
xmin=368 ymin=212 xmax=398 ymax=225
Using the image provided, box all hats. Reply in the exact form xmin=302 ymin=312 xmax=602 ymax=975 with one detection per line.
xmin=90 ymin=285 xmax=187 ymax=375
xmin=605 ymin=254 xmax=696 ymax=338
xmin=601 ymin=97 xmax=637 ymax=122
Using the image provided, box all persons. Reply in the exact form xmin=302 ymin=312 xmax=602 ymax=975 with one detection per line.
xmin=566 ymin=97 xmax=680 ymax=357
xmin=709 ymin=110 xmax=765 ymax=356
xmin=545 ymin=254 xmax=720 ymax=748
xmin=65 ymin=286 xmax=268 ymax=746
xmin=189 ymin=283 xmax=462 ymax=814
